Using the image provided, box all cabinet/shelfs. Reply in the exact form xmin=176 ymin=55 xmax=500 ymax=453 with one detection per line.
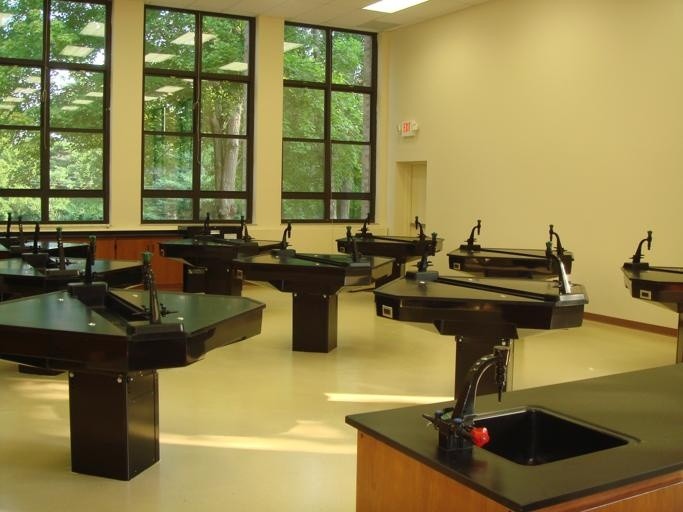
xmin=46 ymin=237 xmax=115 ymax=260
xmin=115 ymin=237 xmax=185 ymax=292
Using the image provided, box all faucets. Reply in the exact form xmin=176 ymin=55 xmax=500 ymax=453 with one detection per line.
xmin=6 ymin=214 xmax=97 ymax=282
xmin=142 ymin=249 xmax=160 ymax=324
xmin=204 ymin=213 xmax=211 ymax=231
xmin=241 ymin=216 xmax=249 ymax=237
xmin=346 ymin=226 xmax=359 ymax=261
xmin=452 ymin=344 xmax=511 ymax=421
xmin=282 ymin=223 xmax=292 ymax=244
xmin=549 ymin=225 xmax=564 ymax=247
xmin=415 ymin=216 xmax=423 ymax=233
xmin=361 ymin=213 xmax=370 ymax=231
xmin=545 ymin=242 xmax=571 ymax=293
xmin=470 ymin=219 xmax=482 ymax=239
xmin=631 ymin=231 xmax=652 ymax=262
xmin=421 ymin=232 xmax=437 ymax=261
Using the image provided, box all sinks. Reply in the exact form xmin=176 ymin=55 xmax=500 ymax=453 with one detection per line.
xmin=473 ymin=406 xmax=641 ymax=467
xmin=294 ymin=254 xmax=348 ymax=268
xmin=73 ymin=285 xmax=145 ymax=336
xmin=373 ymin=236 xmax=412 ymax=243
xmin=435 ymin=275 xmax=551 ymax=303
xmin=206 ymin=237 xmax=236 ymax=247
xmin=480 ymin=247 xmax=547 ymax=258
xmin=649 ymin=267 xmax=683 ymax=274
